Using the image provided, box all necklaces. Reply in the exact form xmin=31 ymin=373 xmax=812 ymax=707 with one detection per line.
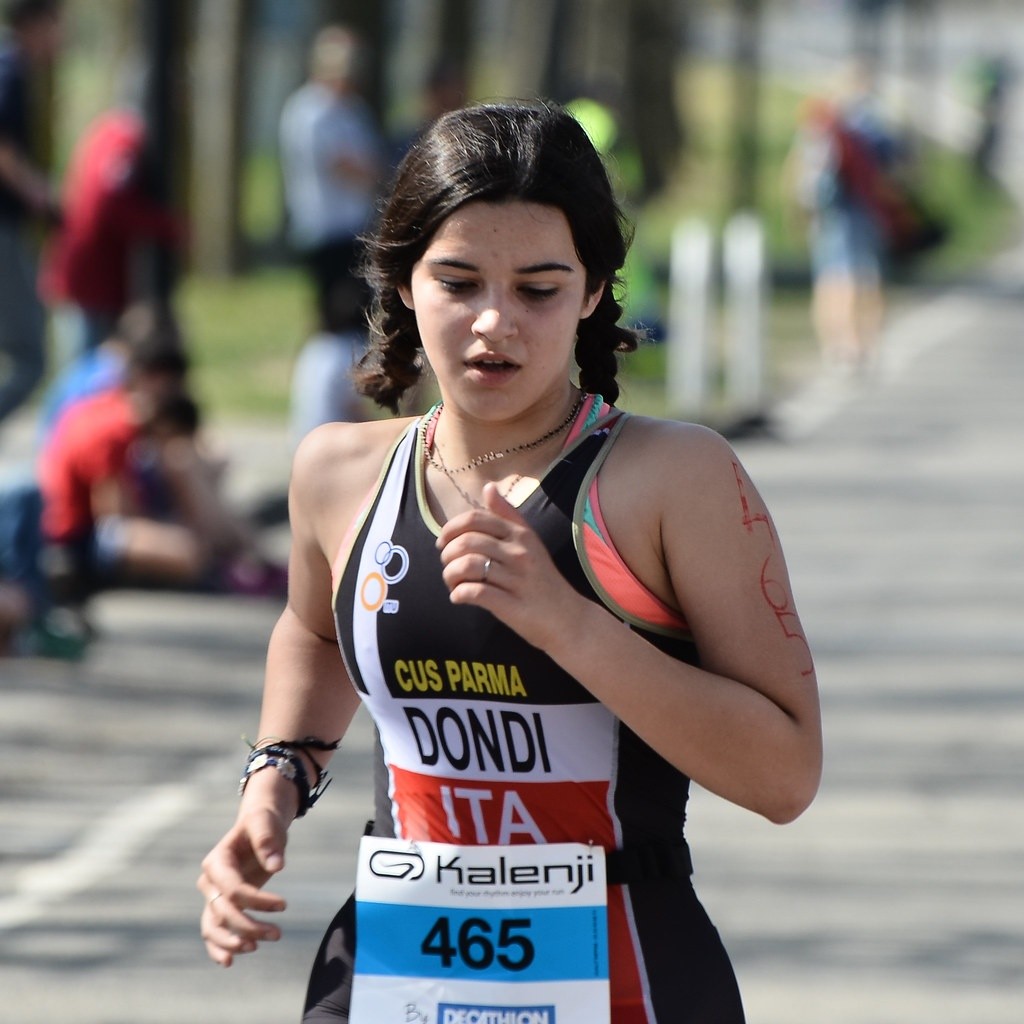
xmin=422 ymin=389 xmax=590 ymax=478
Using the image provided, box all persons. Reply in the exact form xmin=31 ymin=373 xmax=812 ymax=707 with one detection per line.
xmin=196 ymin=96 xmax=825 ymax=1024
xmin=1 ymin=0 xmax=945 ymax=664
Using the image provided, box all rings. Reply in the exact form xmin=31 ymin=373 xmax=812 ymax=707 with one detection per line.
xmin=482 ymin=558 xmax=492 ymax=584
xmin=210 ymin=892 xmax=222 ymax=912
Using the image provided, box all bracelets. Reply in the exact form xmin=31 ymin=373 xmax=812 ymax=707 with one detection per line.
xmin=236 ymin=735 xmax=345 ymax=821
